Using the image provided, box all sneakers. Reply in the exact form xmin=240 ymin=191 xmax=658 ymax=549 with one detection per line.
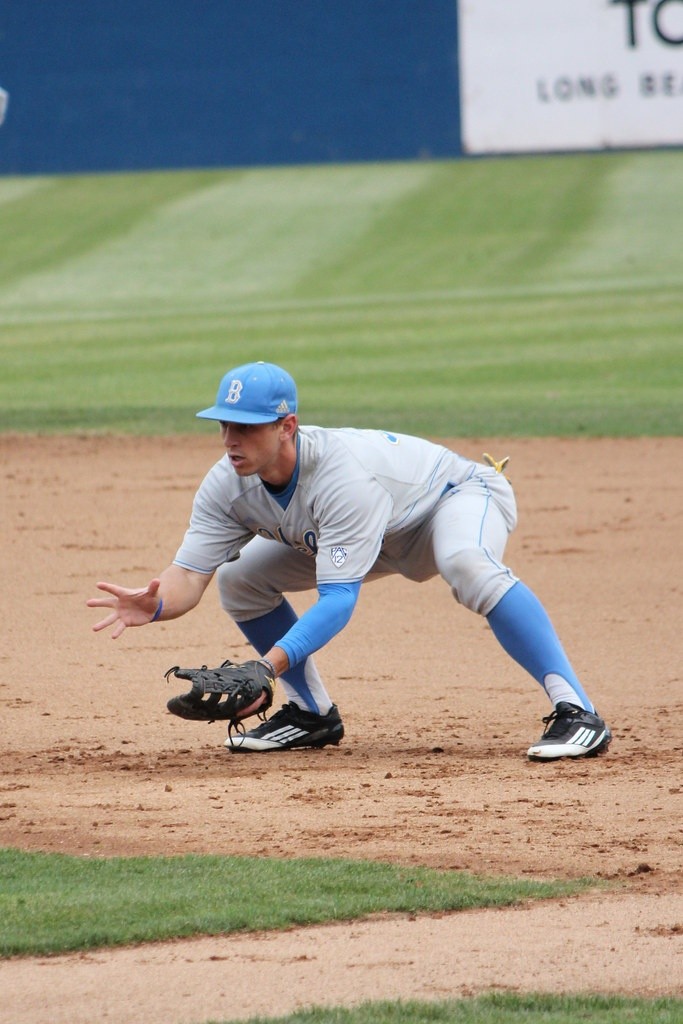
xmin=223 ymin=701 xmax=344 ymax=753
xmin=527 ymin=702 xmax=612 ymax=763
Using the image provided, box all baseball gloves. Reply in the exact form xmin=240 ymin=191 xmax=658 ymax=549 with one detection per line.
xmin=163 ymin=659 xmax=276 ymax=748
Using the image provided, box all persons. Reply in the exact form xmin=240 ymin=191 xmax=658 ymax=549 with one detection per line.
xmin=86 ymin=361 xmax=613 ymax=761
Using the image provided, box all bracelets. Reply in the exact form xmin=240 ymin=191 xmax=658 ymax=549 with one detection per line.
xmin=150 ymin=599 xmax=163 ymax=622
xmin=261 ymin=658 xmax=275 ymax=672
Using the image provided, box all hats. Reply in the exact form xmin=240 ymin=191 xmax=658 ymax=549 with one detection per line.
xmin=196 ymin=361 xmax=298 ymax=423
